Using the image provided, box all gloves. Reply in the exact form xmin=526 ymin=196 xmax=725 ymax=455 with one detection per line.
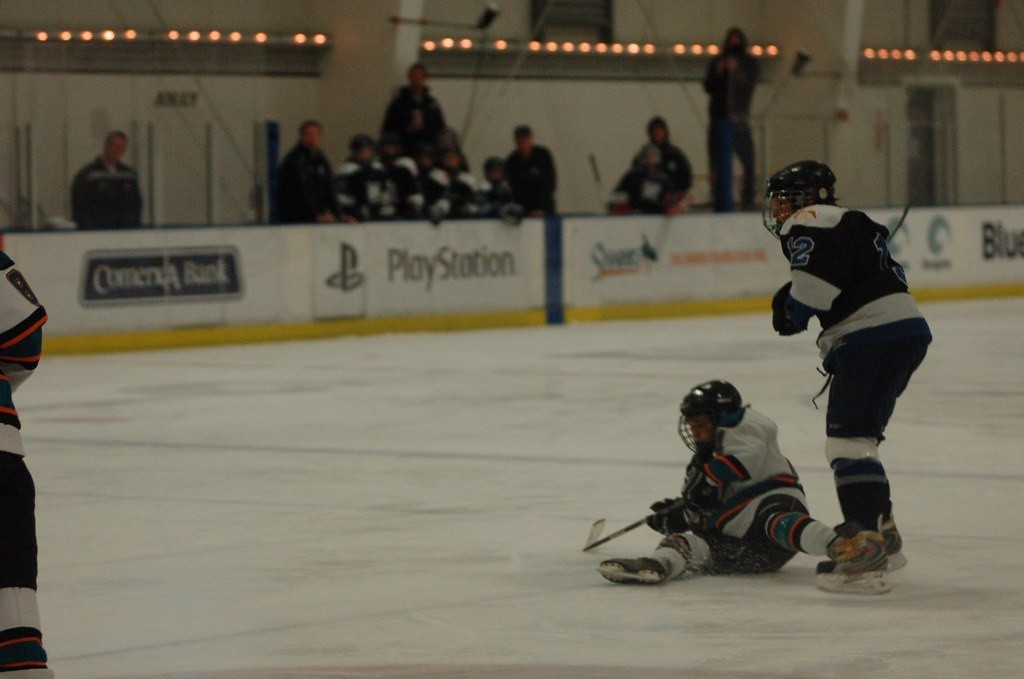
xmin=648 ymin=498 xmax=685 ymax=533
xmin=684 ymin=473 xmax=723 ymax=510
xmin=772 ymin=299 xmax=804 ymax=335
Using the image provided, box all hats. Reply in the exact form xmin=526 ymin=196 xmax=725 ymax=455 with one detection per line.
xmin=350 ymin=134 xmax=377 ymax=150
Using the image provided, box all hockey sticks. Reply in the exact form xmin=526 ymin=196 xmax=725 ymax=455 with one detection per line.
xmin=889 ymin=196 xmax=914 ymax=240
xmin=582 ymin=504 xmax=688 ymax=554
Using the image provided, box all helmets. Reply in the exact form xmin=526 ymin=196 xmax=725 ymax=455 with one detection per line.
xmin=680 ymin=380 xmax=741 ymax=423
xmin=766 ymin=160 xmax=838 ymax=207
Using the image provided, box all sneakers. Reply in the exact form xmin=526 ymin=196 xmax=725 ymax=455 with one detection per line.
xmin=880 ymin=518 xmax=908 ymax=574
xmin=815 ymin=535 xmax=891 ymax=594
xmin=599 ymin=557 xmax=670 ymax=585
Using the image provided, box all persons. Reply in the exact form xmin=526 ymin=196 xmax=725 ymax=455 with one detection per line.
xmin=614 ymin=116 xmax=693 ymax=214
xmin=597 ymin=380 xmax=844 ymax=585
xmin=701 ymin=27 xmax=757 ymax=212
xmin=71 ymin=130 xmax=143 ymax=231
xmin=278 ymin=65 xmax=558 ymax=225
xmin=761 ymin=160 xmax=933 ymax=596
xmin=0 ymin=251 xmax=58 ymax=679
